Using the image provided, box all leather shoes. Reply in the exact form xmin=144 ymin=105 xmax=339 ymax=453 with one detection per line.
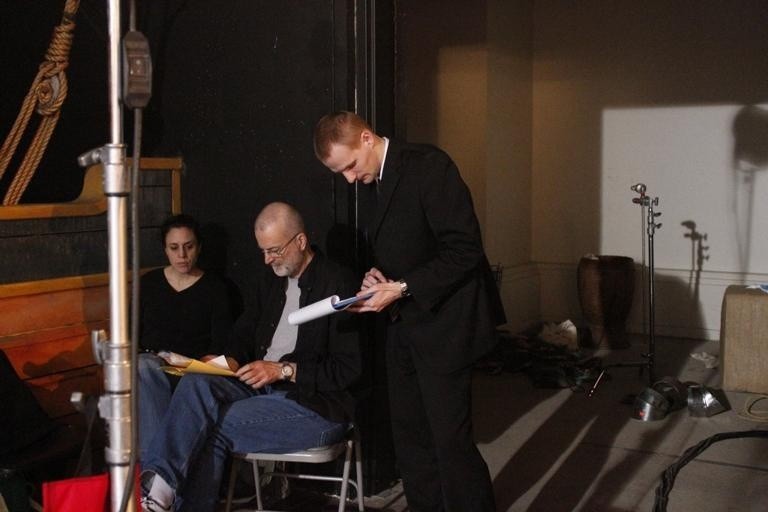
xmin=1 ymin=430 xmax=100 ymax=479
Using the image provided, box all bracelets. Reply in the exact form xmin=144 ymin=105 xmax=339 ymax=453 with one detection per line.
xmin=400 ymin=279 xmax=412 ymax=298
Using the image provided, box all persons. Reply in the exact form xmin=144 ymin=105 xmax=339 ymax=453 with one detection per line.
xmin=312 ymin=110 xmax=507 ymax=511
xmin=128 ymin=215 xmax=234 ymax=471
xmin=142 ymin=201 xmax=379 ymax=511
xmin=0 ymin=351 xmax=60 ymax=511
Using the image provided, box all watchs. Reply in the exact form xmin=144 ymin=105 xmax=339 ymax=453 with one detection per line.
xmin=282 ymin=361 xmax=294 ymax=383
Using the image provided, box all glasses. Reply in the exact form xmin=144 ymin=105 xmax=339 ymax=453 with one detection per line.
xmin=259 ymin=234 xmax=298 ymax=257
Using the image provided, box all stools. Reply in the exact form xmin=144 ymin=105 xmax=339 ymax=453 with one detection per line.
xmin=223 ymin=422 xmax=365 ymax=512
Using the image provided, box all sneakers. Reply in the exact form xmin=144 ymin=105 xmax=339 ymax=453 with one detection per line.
xmin=140 ymin=494 xmax=177 ymax=512
